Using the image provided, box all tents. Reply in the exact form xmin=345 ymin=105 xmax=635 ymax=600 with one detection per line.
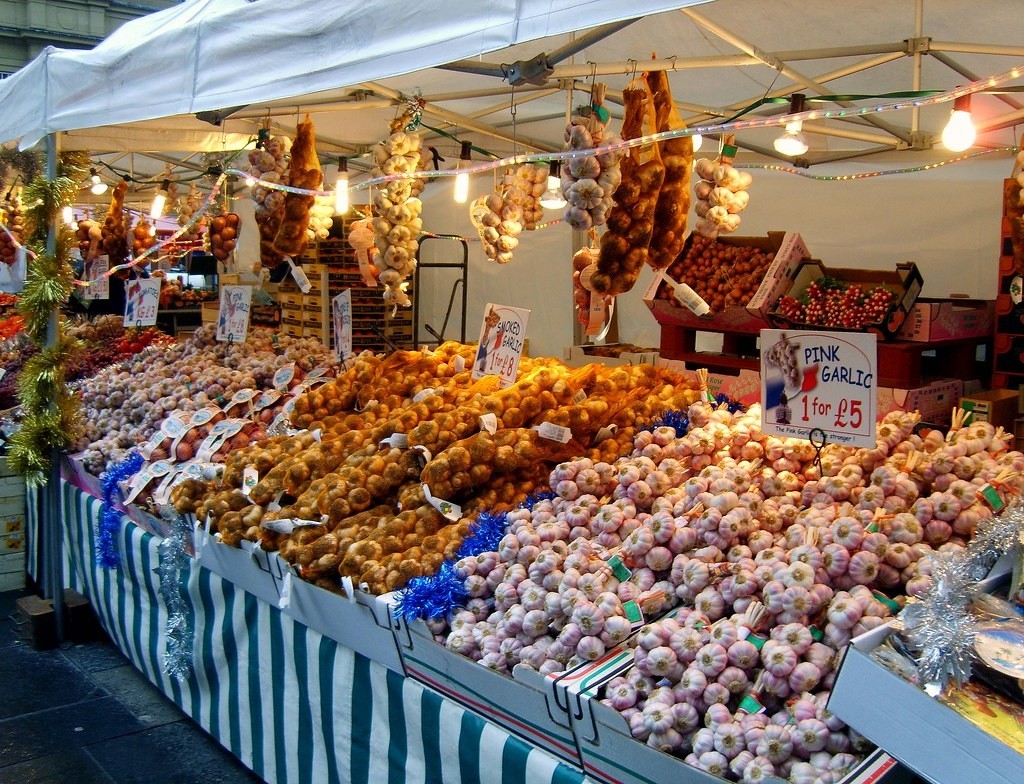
xmin=0 ymin=0 xmax=1024 ymax=639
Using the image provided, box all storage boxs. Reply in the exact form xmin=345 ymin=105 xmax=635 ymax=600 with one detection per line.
xmin=566 ymin=228 xmax=1024 ymax=448
xmin=157 ymin=205 xmax=418 ymax=351
xmin=0 ymin=457 xmax=1024 ymax=784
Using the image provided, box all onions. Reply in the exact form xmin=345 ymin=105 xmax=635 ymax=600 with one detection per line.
xmin=76 ymin=220 xmax=99 ymax=257
xmin=123 ymin=362 xmax=337 ymax=506
xmin=660 ymin=235 xmax=776 ymax=306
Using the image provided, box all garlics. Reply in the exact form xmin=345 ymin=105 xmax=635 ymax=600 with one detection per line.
xmin=172 ymin=341 xmax=705 ymax=593
xmin=154 ymin=183 xmax=204 ymax=242
xmin=100 ymin=188 xmax=131 ymax=279
xmin=0 ymin=196 xmax=31 ymax=267
xmin=429 ymin=407 xmax=1024 ymax=784
xmin=560 ymin=116 xmax=751 ymax=325
xmin=481 ymin=164 xmax=548 ymax=264
xmin=72 ymin=322 xmax=333 ymax=476
xmin=248 ymin=130 xmax=435 ymax=304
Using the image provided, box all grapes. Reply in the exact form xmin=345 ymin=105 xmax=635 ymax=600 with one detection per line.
xmin=0 ymin=336 xmax=122 ymax=412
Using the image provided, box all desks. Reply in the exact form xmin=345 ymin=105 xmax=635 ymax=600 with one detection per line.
xmin=22 ymin=468 xmax=601 ymax=784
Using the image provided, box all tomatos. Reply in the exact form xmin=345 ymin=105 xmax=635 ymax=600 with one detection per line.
xmin=211 ymin=214 xmax=238 ymax=259
xmin=131 ymin=226 xmax=153 ymax=267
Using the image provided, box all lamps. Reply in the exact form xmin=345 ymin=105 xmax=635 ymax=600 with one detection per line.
xmin=539 ymin=161 xmax=568 ymax=209
xmin=89 ymin=168 xmax=108 ymax=195
xmin=774 ymin=94 xmax=809 ymax=156
